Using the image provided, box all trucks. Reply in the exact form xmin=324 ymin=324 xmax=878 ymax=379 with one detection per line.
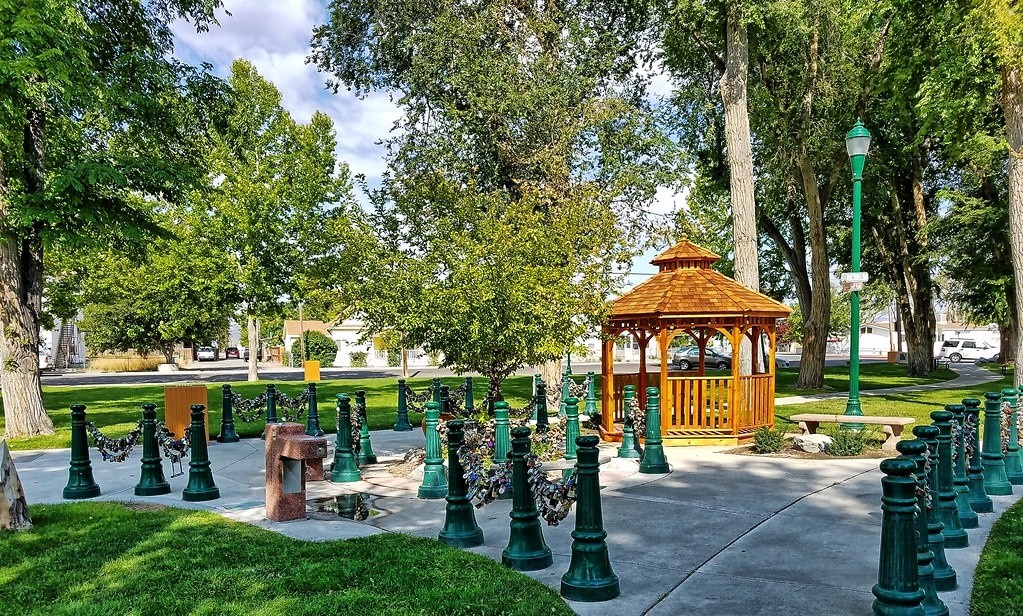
xmin=244 ymin=347 xmax=261 ymax=362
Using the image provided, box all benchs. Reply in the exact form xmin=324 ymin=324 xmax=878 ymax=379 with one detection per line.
xmin=936 ymin=361 xmax=950 ymax=371
xmin=998 ymin=363 xmax=1009 ymax=374
xmin=789 ymin=414 xmax=916 ymax=452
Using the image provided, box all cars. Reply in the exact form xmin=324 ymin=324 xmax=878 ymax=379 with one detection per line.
xmin=727 ymin=351 xmax=790 ymax=368
xmin=940 ymin=339 xmax=1000 ymax=363
xmin=39 ymin=350 xmax=54 ymax=376
xmin=672 ymin=345 xmax=732 ymax=371
xmin=225 ymin=347 xmax=239 ymax=359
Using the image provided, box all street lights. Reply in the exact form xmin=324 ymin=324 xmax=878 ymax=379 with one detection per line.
xmin=840 ymin=117 xmax=872 ymax=430
xmin=298 ymin=299 xmax=305 ymax=368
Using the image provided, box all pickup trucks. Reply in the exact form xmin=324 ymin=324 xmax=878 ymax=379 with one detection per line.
xmin=196 ymin=346 xmax=215 ymax=362
